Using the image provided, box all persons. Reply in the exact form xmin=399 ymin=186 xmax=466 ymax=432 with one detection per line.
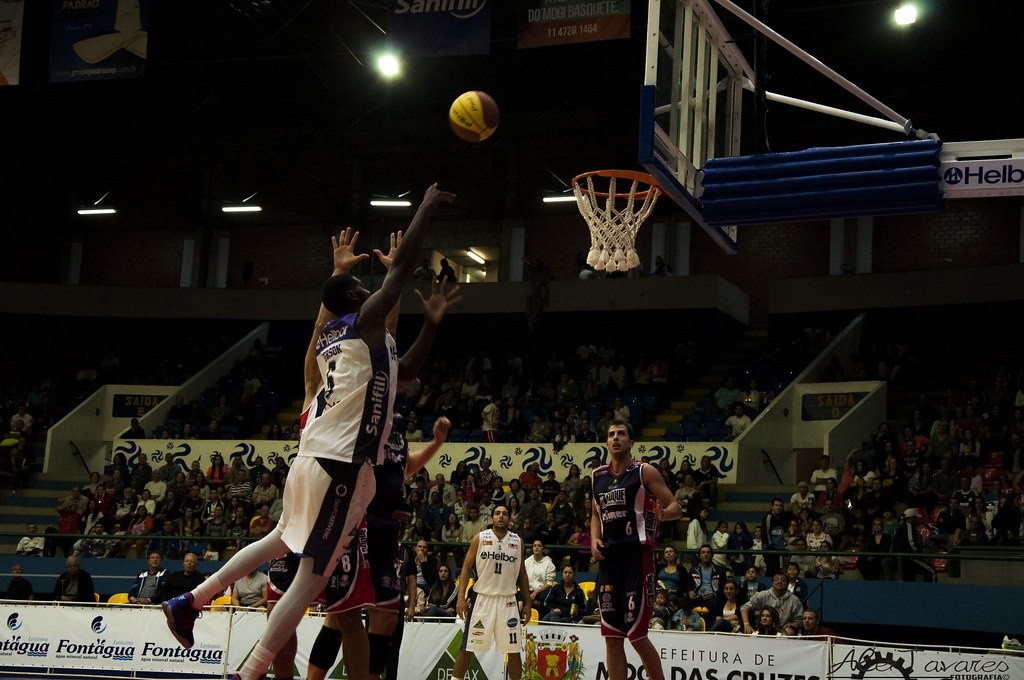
xmin=128 ymin=551 xmax=205 ymax=609
xmin=398 ymin=456 xmax=727 ymax=556
xmin=15 ymin=451 xmax=304 ymax=561
xmin=588 ymin=420 xmax=682 ymax=680
xmin=297 ymin=412 xmax=450 ymax=680
xmin=403 ymin=539 xmax=478 ymax=622
xmin=711 ymin=520 xmax=730 ymax=566
xmin=793 ymin=608 xmax=844 ymax=644
xmin=0 ymin=563 xmax=34 ymax=604
xmin=451 ymin=504 xmax=531 ymax=680
xmin=583 ymin=571 xmax=600 ymax=625
xmin=686 ymin=506 xmax=710 ymax=569
xmin=48 ymin=556 xmax=97 ymax=607
xmin=203 ymin=566 xmax=268 ymax=611
xmin=413 ymin=258 xmax=457 ymax=285
xmin=0 ymin=347 xmax=124 ymax=487
xmin=267 ymin=227 xmax=403 ymax=679
xmin=165 ymin=180 xmax=456 ymax=680
xmin=516 ymin=539 xmax=557 ymax=614
xmin=542 ymin=564 xmax=586 ymax=627
xmin=727 ymin=359 xmax=1024 ymax=581
xmin=647 ymin=545 xmax=808 ymax=635
xmin=124 ymin=337 xmax=800 ymax=443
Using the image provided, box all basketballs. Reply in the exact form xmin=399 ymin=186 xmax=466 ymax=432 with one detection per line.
xmin=448 ymin=90 xmax=501 ymax=143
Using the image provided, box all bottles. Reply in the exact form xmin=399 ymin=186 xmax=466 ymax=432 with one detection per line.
xmin=570 ymin=597 xmax=575 ymax=619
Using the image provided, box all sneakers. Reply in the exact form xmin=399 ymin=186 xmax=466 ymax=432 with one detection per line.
xmin=162 ymin=592 xmax=203 ymax=649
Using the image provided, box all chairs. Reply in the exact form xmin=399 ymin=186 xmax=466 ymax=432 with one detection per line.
xmin=93 ymin=481 xmax=766 ymax=627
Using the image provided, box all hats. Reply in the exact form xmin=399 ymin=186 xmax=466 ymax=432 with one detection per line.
xmin=903 ymin=508 xmax=922 ymax=519
